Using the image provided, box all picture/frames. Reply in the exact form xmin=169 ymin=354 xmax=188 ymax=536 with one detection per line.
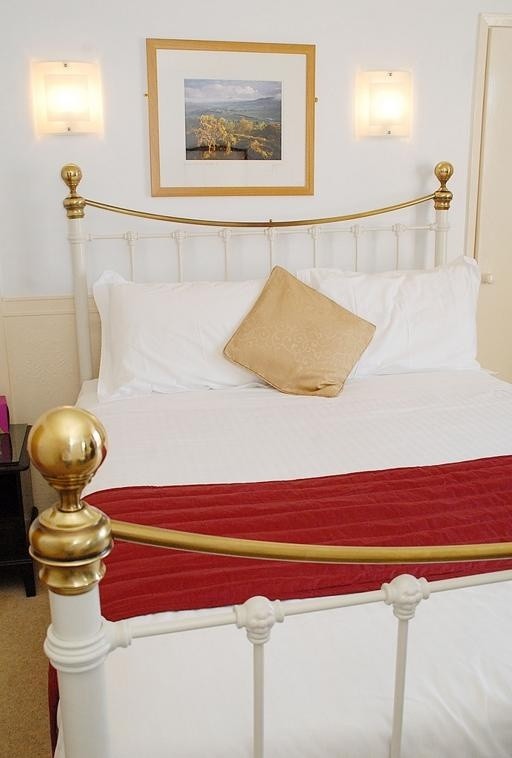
xmin=144 ymin=35 xmax=321 ymax=200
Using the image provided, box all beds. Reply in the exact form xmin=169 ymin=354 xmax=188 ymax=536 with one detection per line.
xmin=21 ymin=160 xmax=508 ymax=757
